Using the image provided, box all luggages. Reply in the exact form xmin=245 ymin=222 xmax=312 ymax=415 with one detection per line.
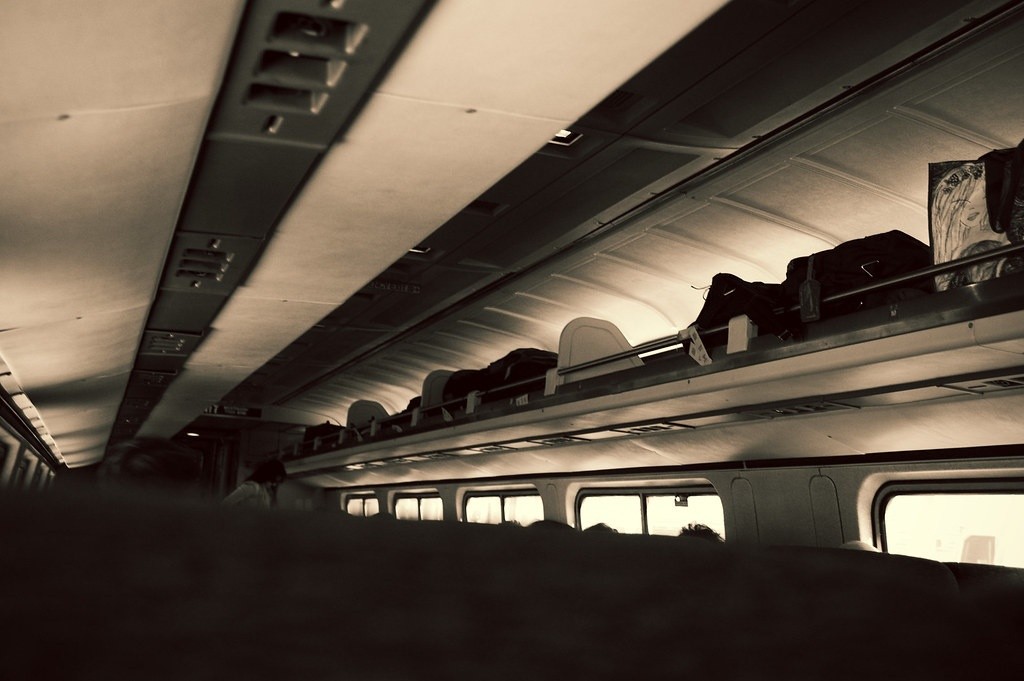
xmin=302 ymin=421 xmax=346 ymax=449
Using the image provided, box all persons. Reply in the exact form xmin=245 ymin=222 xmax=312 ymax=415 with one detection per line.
xmin=223 ymin=459 xmax=313 ymax=512
xmin=95 ymin=436 xmax=204 ymax=503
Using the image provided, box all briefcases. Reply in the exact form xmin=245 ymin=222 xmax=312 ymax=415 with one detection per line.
xmin=780 ymin=230 xmax=930 ymax=324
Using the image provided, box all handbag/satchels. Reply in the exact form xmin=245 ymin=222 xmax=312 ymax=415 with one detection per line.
xmin=928 ymin=138 xmax=1024 ymax=292
xmin=680 ymin=272 xmax=798 ymax=367
xmin=442 ymin=348 xmax=559 ymax=408
